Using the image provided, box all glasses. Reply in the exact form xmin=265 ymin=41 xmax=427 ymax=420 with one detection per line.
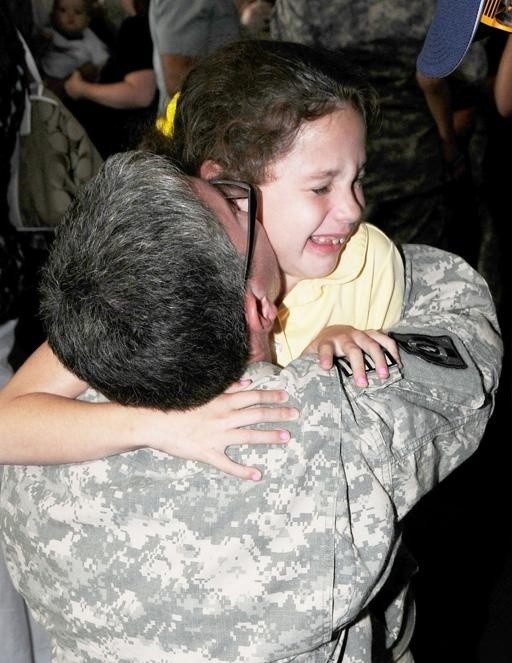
xmin=208 ymin=178 xmax=257 ymax=291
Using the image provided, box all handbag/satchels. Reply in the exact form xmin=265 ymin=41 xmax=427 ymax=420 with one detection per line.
xmin=10 ymin=77 xmax=107 ymax=234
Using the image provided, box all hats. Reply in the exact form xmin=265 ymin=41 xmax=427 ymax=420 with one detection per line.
xmin=417 ymin=0 xmax=483 ymax=79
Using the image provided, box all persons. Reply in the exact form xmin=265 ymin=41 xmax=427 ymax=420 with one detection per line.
xmin=0 ymin=0 xmax=508 ymax=339
xmin=2 ymin=42 xmax=405 ymax=480
xmin=0 ymin=149 xmax=502 ymax=663
